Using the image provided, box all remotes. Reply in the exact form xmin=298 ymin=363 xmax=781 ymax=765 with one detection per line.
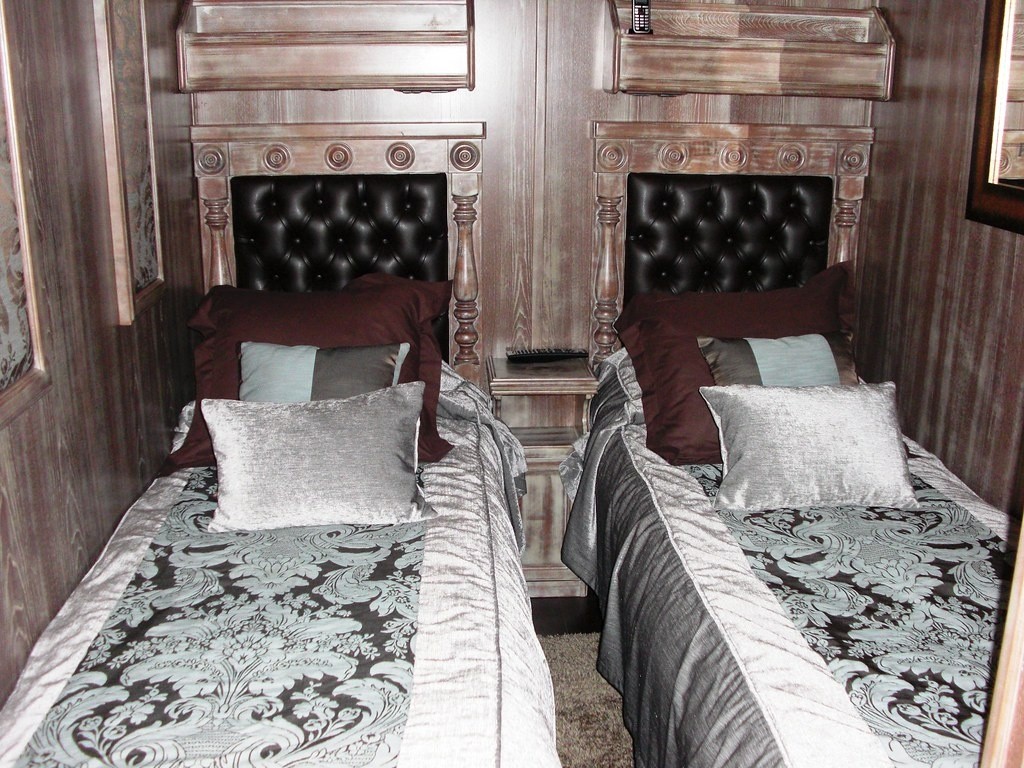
xmin=506 ymin=349 xmax=589 ymax=362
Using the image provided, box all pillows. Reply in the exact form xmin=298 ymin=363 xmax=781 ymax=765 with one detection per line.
xmin=238 ymin=340 xmax=412 ymax=403
xmin=699 ymin=380 xmax=920 ymax=512
xmin=201 ymin=381 xmax=438 ymax=533
xmin=696 ymin=329 xmax=859 ymax=386
xmin=611 ymin=260 xmax=857 ymax=467
xmin=157 ymin=271 xmax=455 ymax=463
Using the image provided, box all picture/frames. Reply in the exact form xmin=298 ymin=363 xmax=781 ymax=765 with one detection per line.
xmin=92 ymin=1 xmax=164 ymax=327
xmin=0 ymin=1 xmax=53 ymax=431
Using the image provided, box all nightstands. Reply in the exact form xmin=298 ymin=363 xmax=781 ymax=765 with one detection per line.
xmin=488 ymin=357 xmax=599 ymax=598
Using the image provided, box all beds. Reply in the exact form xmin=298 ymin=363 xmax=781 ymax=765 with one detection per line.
xmin=556 ymin=121 xmax=1023 ymax=768
xmin=1 ymin=111 xmax=564 ymax=767
